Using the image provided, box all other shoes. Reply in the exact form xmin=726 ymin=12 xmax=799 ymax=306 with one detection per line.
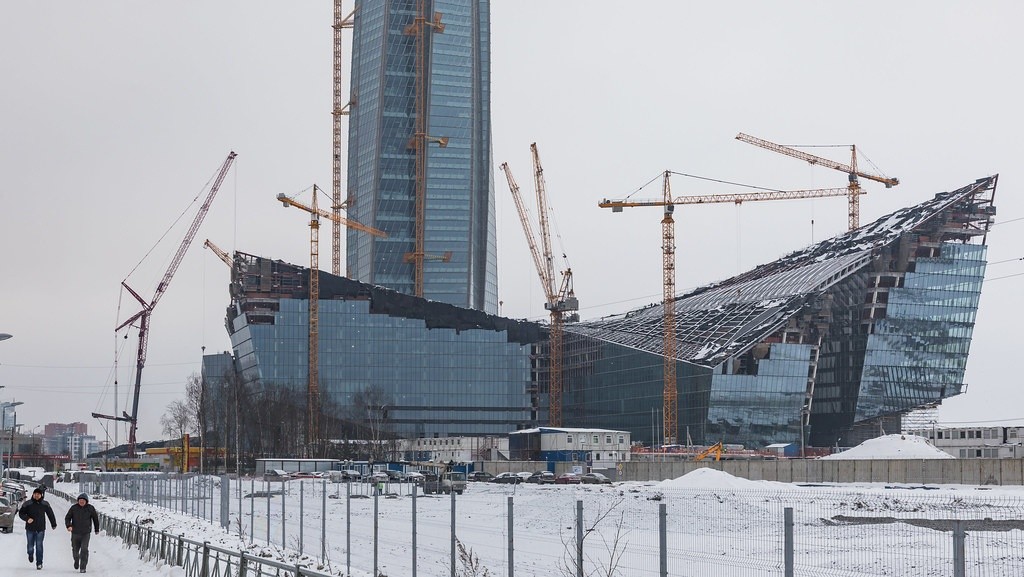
xmin=37 ymin=564 xmax=42 ymax=569
xmin=80 ymin=567 xmax=85 ymax=573
xmin=29 ymin=554 xmax=33 ymax=563
xmin=74 ymin=559 xmax=79 ymax=569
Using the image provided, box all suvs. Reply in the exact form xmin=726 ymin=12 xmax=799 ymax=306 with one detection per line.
xmin=467 ymin=471 xmax=494 ymax=482
xmin=526 ymin=471 xmax=556 ymax=484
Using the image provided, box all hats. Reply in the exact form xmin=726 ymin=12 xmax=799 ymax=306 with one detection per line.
xmin=77 ymin=493 xmax=88 ymax=504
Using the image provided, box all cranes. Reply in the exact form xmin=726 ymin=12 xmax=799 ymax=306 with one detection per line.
xmin=92 ymin=150 xmax=238 ymax=458
xmin=735 ymin=132 xmax=899 ymax=232
xmin=405 ymin=0 xmax=453 ymax=298
xmin=499 ymin=141 xmax=580 ymax=428
xmin=598 ymin=169 xmax=867 ymax=444
xmin=276 ymin=183 xmax=387 ymax=456
xmin=332 ymin=0 xmax=359 ymax=276
xmin=202 ymin=239 xmax=233 ymax=269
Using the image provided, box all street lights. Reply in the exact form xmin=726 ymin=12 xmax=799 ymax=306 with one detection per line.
xmin=0 ymin=401 xmax=25 ymax=486
xmin=0 ymin=333 xmax=13 ymax=340
xmin=7 ymin=424 xmax=24 ymax=482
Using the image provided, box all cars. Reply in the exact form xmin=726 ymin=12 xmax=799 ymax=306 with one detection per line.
xmin=0 ymin=482 xmax=27 ymax=514
xmin=365 ymin=472 xmax=388 ymax=482
xmin=405 ymin=472 xmax=424 ymax=480
xmin=580 ymin=473 xmax=611 ymax=484
xmin=516 ymin=472 xmax=533 ymax=481
xmin=312 ymin=472 xmax=323 ymax=478
xmin=290 ymin=472 xmax=316 ymax=479
xmin=488 ymin=472 xmax=523 ymax=484
xmin=555 ymin=473 xmax=580 ymax=484
xmin=0 ymin=496 xmax=13 ymax=533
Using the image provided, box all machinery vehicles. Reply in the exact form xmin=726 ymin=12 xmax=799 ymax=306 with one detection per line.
xmin=370 ymin=458 xmax=467 ymax=494
xmin=690 ymin=440 xmax=722 ymax=462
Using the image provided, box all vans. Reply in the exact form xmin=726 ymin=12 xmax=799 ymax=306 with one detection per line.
xmin=381 ymin=470 xmax=400 ymax=482
xmin=420 ymin=470 xmax=437 ymax=481
xmin=263 ymin=469 xmax=290 ymax=482
xmin=324 ymin=471 xmax=344 ymax=482
xmin=341 ymin=470 xmax=362 ymax=480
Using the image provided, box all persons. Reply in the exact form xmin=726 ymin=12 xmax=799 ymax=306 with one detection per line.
xmin=39 ymin=484 xmax=47 ymax=499
xmin=19 ymin=488 xmax=57 ymax=570
xmin=65 ymin=493 xmax=99 ymax=573
